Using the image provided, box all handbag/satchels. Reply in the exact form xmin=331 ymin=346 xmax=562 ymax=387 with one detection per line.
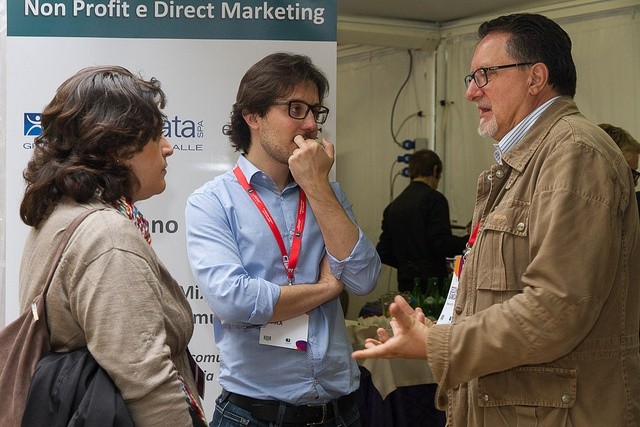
xmin=0 ymin=207 xmax=108 ymax=427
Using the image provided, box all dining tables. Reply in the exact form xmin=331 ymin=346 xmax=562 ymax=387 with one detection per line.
xmin=344 ymin=318 xmax=447 ymax=427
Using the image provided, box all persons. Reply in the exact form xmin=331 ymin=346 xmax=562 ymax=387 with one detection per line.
xmin=351 ymin=12 xmax=640 ymax=427
xmin=185 ymin=51 xmax=381 ymax=427
xmin=17 ymin=65 xmax=208 ymax=427
xmin=376 ymin=149 xmax=471 ymax=298
xmin=597 ymin=123 xmax=640 ymax=189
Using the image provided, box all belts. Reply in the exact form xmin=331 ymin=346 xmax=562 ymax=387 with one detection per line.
xmin=225 ymin=387 xmax=360 ymax=425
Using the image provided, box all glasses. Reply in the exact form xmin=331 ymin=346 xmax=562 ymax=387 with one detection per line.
xmin=464 ymin=59 xmax=538 ymax=88
xmin=271 ymin=99 xmax=329 ymax=123
xmin=631 ymin=169 xmax=640 ymax=184
